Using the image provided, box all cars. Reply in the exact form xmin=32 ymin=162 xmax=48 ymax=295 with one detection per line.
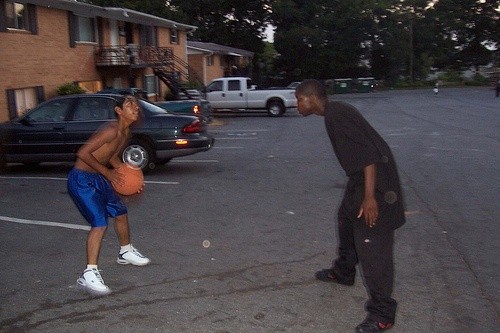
xmin=0 ymin=94 xmax=217 ymax=176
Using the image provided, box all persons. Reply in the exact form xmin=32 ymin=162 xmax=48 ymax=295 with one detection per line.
xmin=294 ymin=80 xmax=407 ymax=333
xmin=66 ymin=88 xmax=150 ymax=293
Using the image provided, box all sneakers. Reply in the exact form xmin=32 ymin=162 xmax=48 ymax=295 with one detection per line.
xmin=356 ymin=317 xmax=393 ymax=333
xmin=76 ymin=268 xmax=109 ymax=291
xmin=315 ymin=269 xmax=341 ymax=283
xmin=116 ymin=247 xmax=151 ymax=266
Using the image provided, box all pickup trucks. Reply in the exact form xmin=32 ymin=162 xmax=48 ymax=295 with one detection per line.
xmin=93 ymin=88 xmax=213 ymax=125
xmin=177 ymin=76 xmax=299 ymax=118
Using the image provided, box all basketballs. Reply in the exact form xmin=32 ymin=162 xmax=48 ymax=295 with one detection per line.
xmin=111 ymin=162 xmax=144 ymax=195
